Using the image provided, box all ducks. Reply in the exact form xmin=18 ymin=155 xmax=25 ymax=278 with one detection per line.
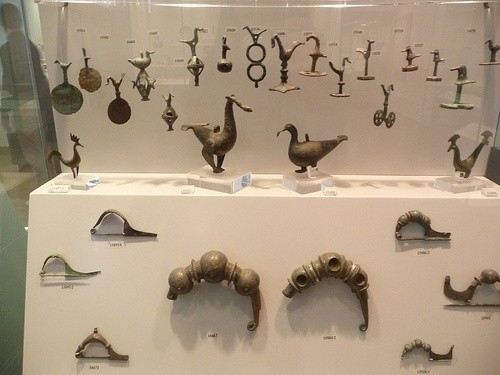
xmin=277 ymin=124 xmax=349 ymax=172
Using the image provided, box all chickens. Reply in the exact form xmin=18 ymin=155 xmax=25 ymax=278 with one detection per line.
xmin=447 ymin=130 xmax=493 ymax=177
xmin=48 ymin=134 xmax=84 ymax=179
